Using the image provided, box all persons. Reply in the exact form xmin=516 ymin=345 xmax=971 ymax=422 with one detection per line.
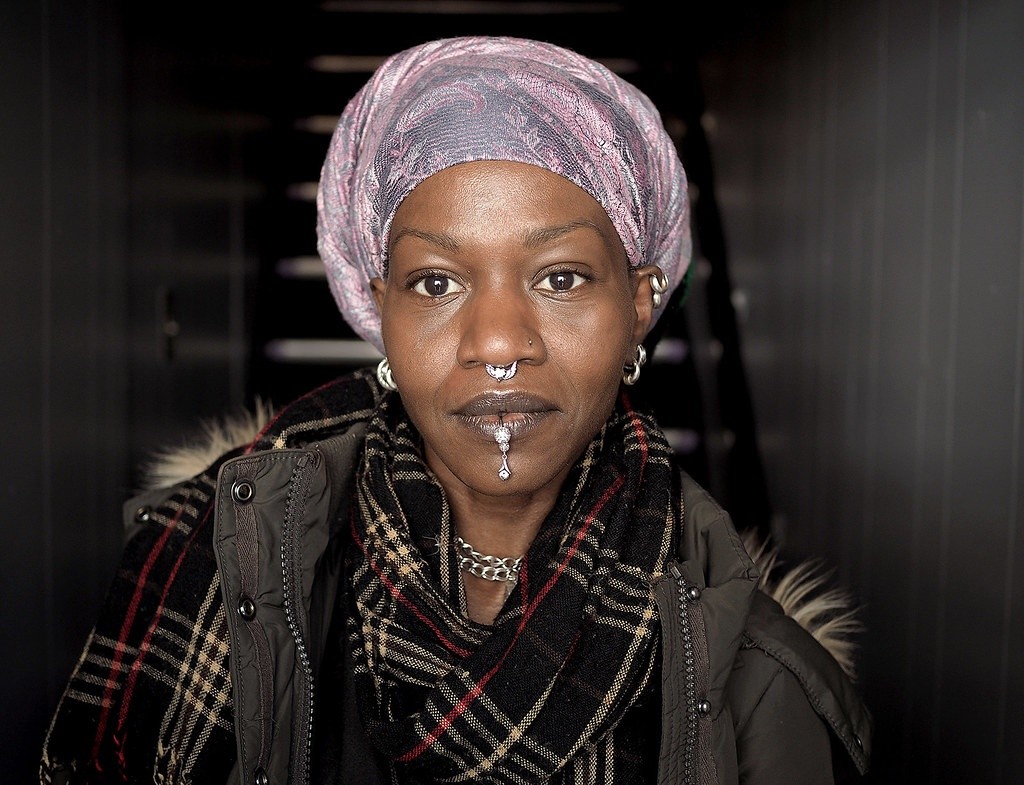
xmin=34 ymin=34 xmax=879 ymax=784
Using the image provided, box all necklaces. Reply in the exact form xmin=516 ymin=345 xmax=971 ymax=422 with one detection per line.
xmin=456 ymin=536 xmax=525 ymax=584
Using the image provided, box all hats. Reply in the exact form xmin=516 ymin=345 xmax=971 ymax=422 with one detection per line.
xmin=314 ymin=37 xmax=694 ymax=359
